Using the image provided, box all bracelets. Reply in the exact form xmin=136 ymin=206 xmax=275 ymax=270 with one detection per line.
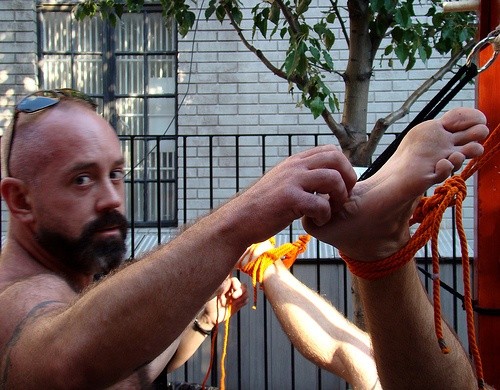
xmin=191 ymin=319 xmax=213 ymax=335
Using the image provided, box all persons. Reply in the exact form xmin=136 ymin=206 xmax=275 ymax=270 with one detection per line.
xmin=1 ymin=98 xmax=356 ymax=390
xmin=236 ymin=107 xmax=490 ymax=390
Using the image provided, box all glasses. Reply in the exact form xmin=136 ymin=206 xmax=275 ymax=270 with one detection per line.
xmin=6 ymin=88 xmax=100 ymax=177
